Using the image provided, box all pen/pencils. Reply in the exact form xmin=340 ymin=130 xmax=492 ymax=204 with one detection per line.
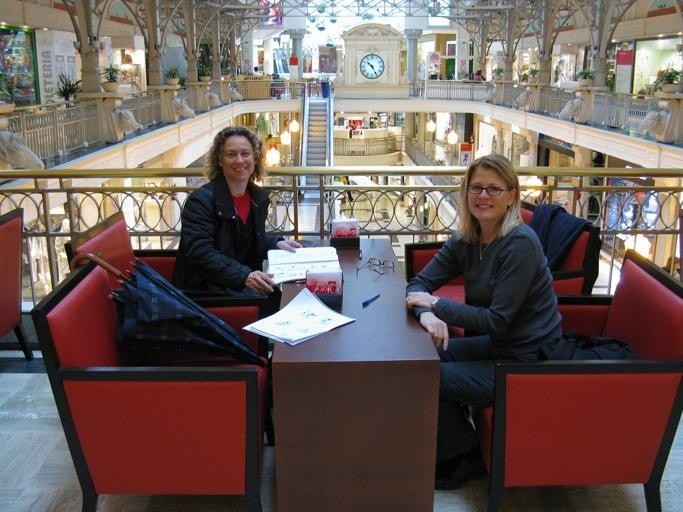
xmin=363 ymin=295 xmax=379 ymax=307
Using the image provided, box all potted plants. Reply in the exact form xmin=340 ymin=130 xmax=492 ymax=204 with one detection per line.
xmin=526 ymin=69 xmax=539 ymax=83
xmin=575 ymin=71 xmax=595 ymax=87
xmin=492 ymin=69 xmax=502 ymax=82
xmin=654 ymin=69 xmax=681 ymax=93
xmin=97 ymin=65 xmax=121 ymax=93
xmin=197 ymin=67 xmax=211 ymax=82
xmin=166 ymin=67 xmax=180 ymax=85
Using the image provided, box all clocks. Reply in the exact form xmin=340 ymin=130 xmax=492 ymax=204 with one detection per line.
xmin=359 ymin=54 xmax=384 ymax=79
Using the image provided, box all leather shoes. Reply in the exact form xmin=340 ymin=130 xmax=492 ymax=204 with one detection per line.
xmin=435 ymin=452 xmax=482 ymax=490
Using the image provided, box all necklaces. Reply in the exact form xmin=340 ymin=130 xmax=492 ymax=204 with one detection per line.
xmin=478 ymin=230 xmax=499 ymax=262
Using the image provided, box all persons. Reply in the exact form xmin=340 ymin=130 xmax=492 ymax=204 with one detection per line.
xmin=473 ymin=69 xmax=486 ymax=84
xmin=404 ymin=154 xmax=565 ymax=491
xmin=253 ymin=66 xmax=261 ymax=75
xmin=345 ymin=120 xmax=355 ymax=138
xmin=171 ymin=126 xmax=303 ymax=352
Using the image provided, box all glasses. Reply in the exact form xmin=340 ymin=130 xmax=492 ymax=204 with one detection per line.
xmin=468 ymin=185 xmax=511 ymax=196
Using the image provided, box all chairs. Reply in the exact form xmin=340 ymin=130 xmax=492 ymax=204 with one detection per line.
xmin=30 ymin=251 xmax=266 ymax=511
xmin=0 ymin=207 xmax=34 ymax=361
xmin=461 ymin=248 xmax=683 ymax=512
xmin=405 ymin=201 xmax=602 ymax=337
xmin=64 ymin=210 xmax=259 ymax=296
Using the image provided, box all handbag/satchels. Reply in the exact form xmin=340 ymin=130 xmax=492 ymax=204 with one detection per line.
xmin=538 ymin=331 xmax=635 ymax=360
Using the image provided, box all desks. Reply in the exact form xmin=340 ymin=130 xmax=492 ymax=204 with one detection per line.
xmin=270 ymin=237 xmax=441 ymax=511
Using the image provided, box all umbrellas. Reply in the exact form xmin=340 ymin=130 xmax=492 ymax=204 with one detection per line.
xmin=64 ymin=251 xmax=269 ymax=371
